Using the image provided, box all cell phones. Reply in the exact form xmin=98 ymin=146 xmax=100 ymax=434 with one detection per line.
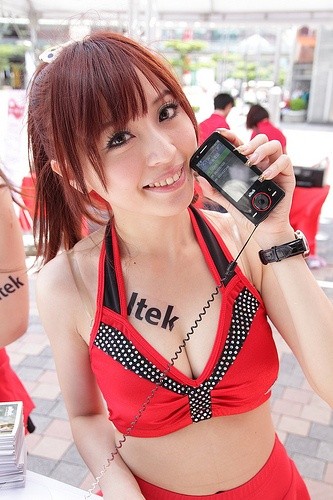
xmin=189 ymin=131 xmax=285 ymax=225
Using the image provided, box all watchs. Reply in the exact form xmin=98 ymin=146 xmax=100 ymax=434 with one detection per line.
xmin=258 ymin=228 xmax=311 ymax=264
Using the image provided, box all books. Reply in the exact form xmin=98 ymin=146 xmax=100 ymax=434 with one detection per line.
xmin=0 ymin=400 xmax=26 ymax=490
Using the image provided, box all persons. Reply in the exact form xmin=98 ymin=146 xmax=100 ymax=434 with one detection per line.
xmin=29 ymin=33 xmax=333 ymax=500
xmin=195 ymin=92 xmax=236 ymax=155
xmin=0 ymin=165 xmax=35 ymax=446
xmin=246 ymin=103 xmax=287 ymax=157
